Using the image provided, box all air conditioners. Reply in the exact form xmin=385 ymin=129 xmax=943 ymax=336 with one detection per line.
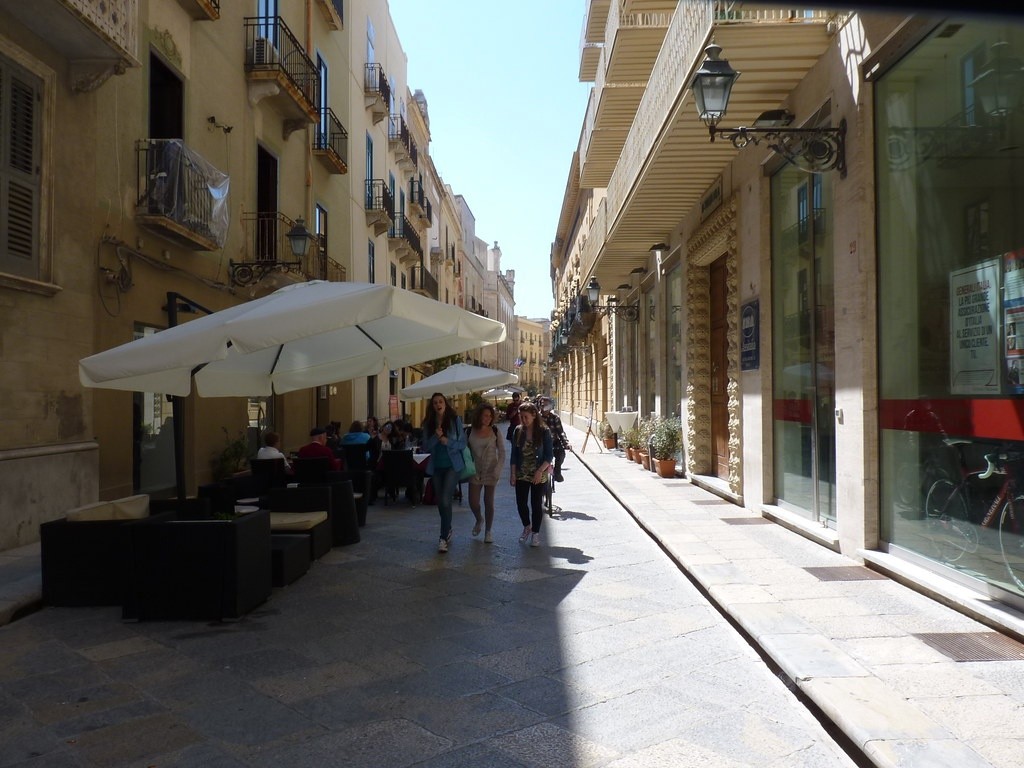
xmin=254 ymin=38 xmax=280 ymax=70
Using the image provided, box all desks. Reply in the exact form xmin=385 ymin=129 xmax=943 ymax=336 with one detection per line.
xmin=412 ymin=453 xmax=462 ymax=504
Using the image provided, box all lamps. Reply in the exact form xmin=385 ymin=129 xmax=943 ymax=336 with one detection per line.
xmin=687 ymin=35 xmax=848 ymax=180
xmin=629 ymin=267 xmax=647 ymax=275
xmin=615 ymin=284 xmax=632 ymax=291
xmin=606 ymin=298 xmax=620 ymax=303
xmin=207 ymin=115 xmax=233 ymax=134
xmin=648 ymin=243 xmax=670 ymax=252
xmin=230 ymin=215 xmax=315 ymax=296
xmin=586 ymin=276 xmax=639 ymax=325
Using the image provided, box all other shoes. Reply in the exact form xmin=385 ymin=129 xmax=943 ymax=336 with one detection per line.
xmin=472 ymin=519 xmax=484 ymax=536
xmin=555 ymin=474 xmax=563 ymax=482
xmin=485 ymin=530 xmax=493 ymax=543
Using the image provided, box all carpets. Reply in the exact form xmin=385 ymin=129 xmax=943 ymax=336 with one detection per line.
xmin=910 ymin=632 xmax=1024 ymax=662
xmin=689 ymin=499 xmax=735 ymax=506
xmin=663 ymin=482 xmax=697 ymax=488
xmin=720 ymin=517 xmax=776 ymax=525
xmin=802 ymin=565 xmax=889 ymax=581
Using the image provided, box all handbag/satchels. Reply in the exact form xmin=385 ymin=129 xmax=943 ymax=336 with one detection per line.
xmin=458 ymin=424 xmax=476 ymax=480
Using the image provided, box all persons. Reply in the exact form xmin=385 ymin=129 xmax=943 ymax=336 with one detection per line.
xmin=328 ymin=420 xmax=371 ymax=469
xmin=505 ymin=392 xmax=572 ymax=548
xmin=364 ymin=416 xmax=420 ymax=508
xmin=421 ymin=392 xmax=468 ymax=553
xmin=259 ymin=430 xmax=295 ymax=479
xmin=463 ymin=404 xmax=505 ymax=543
xmin=297 ymin=427 xmax=341 ymax=473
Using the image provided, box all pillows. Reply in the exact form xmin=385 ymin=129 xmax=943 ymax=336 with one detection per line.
xmin=110 ymin=493 xmax=150 ymax=520
xmin=65 ymin=501 xmax=113 ymax=521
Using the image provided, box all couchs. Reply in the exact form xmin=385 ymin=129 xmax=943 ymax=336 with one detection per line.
xmin=39 ymin=463 xmax=373 ymax=621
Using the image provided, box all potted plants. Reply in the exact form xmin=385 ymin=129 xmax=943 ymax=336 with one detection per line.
xmin=630 ymin=415 xmax=650 ymax=464
xmin=639 ymin=414 xmax=666 ymax=470
xmin=600 ymin=420 xmax=616 ymax=450
xmin=206 ymin=423 xmax=252 ymax=498
xmin=621 ymin=428 xmax=636 ymax=461
xmin=652 ymin=411 xmax=683 ymax=478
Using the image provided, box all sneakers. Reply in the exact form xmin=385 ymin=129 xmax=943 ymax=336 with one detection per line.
xmin=529 ymin=533 xmax=539 ymax=546
xmin=520 ymin=524 xmax=533 ymax=542
xmin=438 ymin=539 xmax=448 ymax=551
xmin=447 ymin=530 xmax=452 ymax=540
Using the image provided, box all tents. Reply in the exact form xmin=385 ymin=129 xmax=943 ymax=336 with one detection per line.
xmin=481 ymin=387 xmax=514 ymax=405
xmin=399 ymin=362 xmax=518 ymax=403
xmin=76 ymin=279 xmax=506 ymax=398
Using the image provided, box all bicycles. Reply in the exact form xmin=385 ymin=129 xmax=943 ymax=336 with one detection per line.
xmin=925 ymin=454 xmax=1024 ymax=592
xmin=543 ymin=446 xmax=570 ymax=518
xmin=896 ymin=444 xmax=950 ymax=509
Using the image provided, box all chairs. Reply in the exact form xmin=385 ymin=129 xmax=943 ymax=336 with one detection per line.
xmin=294 ymin=458 xmax=331 ymax=482
xmin=381 ymin=449 xmax=424 ymax=508
xmin=341 ymin=443 xmax=375 ymax=505
xmin=250 ymin=458 xmax=286 ymax=492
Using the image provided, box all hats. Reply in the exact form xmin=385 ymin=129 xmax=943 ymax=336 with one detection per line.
xmin=310 ymin=428 xmax=327 ymax=436
